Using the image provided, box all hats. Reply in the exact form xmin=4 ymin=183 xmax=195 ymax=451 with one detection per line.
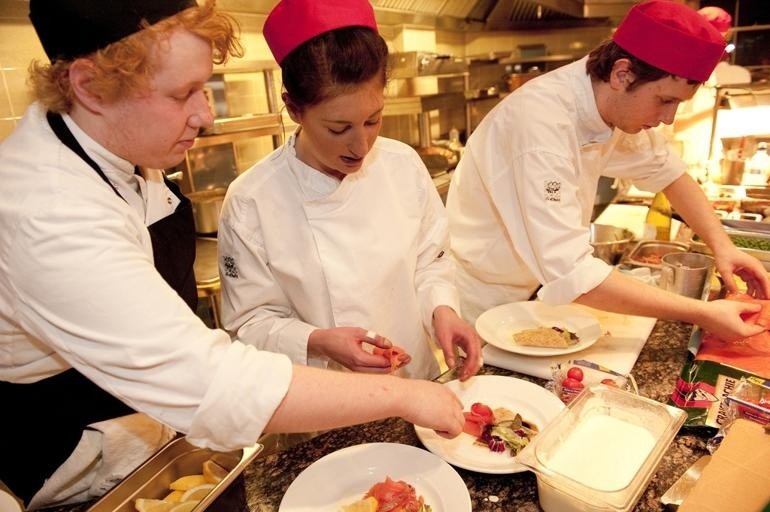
xmin=263 ymin=0 xmax=378 ymax=65
xmin=697 ymin=7 xmax=732 ymax=32
xmin=613 ymin=0 xmax=726 ymax=81
xmin=29 ymin=0 xmax=198 ymax=64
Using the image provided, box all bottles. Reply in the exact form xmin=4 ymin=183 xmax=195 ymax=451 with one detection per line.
xmin=747 ymin=143 xmax=770 ymax=185
xmin=643 ymin=188 xmax=670 ymax=240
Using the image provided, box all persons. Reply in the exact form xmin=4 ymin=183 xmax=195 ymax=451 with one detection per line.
xmin=652 ymin=6 xmax=754 ymax=161
xmin=217 ymin=0 xmax=482 ymax=446
xmin=447 ymin=1 xmax=770 ymax=359
xmin=0 ymin=1 xmax=466 ymax=511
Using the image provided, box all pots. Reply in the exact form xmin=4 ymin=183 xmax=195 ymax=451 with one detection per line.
xmin=187 ymin=190 xmax=226 ymax=236
xmin=418 ymin=54 xmax=450 ymax=74
xmin=589 ymin=223 xmax=631 ymax=269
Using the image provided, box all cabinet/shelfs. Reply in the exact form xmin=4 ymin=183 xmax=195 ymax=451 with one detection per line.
xmin=180 ymin=60 xmax=423 ymax=289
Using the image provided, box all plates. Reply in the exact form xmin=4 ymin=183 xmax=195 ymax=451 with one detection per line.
xmin=279 ymin=443 xmax=473 ymax=512
xmin=413 ymin=375 xmax=567 ymax=475
xmin=475 ymin=301 xmax=601 ymax=356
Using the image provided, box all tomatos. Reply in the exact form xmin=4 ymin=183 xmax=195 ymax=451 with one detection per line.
xmin=601 ymin=379 xmax=616 ymax=387
xmin=566 ymin=366 xmax=583 ymax=382
xmin=561 ymin=378 xmax=583 ymax=388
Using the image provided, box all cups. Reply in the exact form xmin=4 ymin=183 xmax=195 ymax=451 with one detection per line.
xmin=661 ymin=253 xmax=712 ymax=309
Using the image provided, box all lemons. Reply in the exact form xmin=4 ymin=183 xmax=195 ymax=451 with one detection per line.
xmin=341 ymin=497 xmax=378 ymax=512
xmin=132 ymin=459 xmax=229 ymax=512
